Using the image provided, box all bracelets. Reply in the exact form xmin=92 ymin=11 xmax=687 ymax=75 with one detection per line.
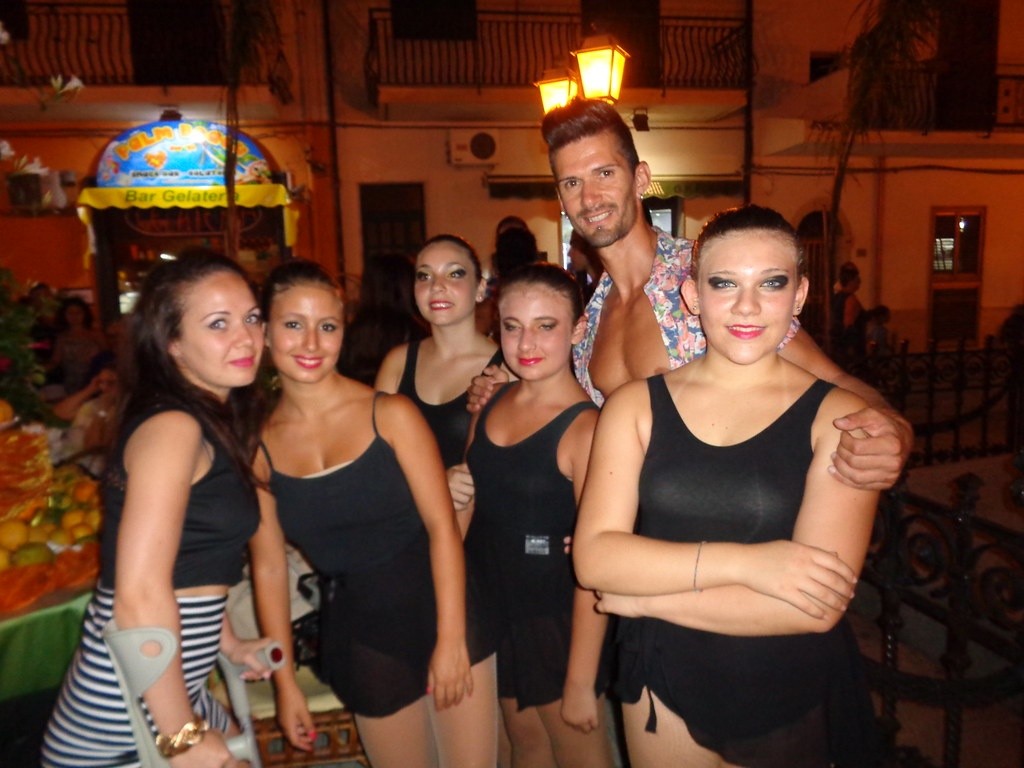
xmin=97 ymin=411 xmax=107 ymax=417
xmin=156 ymin=712 xmax=210 ymax=758
xmin=693 ymin=541 xmax=706 ymax=589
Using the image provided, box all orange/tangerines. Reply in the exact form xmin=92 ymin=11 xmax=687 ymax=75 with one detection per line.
xmin=0 ymin=470 xmax=102 ymax=573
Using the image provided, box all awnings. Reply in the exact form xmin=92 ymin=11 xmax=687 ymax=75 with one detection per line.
xmin=74 ymin=183 xmax=300 ymax=271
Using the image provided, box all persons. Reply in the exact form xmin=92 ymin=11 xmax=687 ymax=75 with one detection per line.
xmin=572 ymin=202 xmax=878 ymax=768
xmin=38 ymin=246 xmax=266 ymax=768
xmin=30 ymin=200 xmax=657 ymax=454
xmin=831 ymin=262 xmax=888 ymax=346
xmin=375 ymin=234 xmax=523 ymax=768
xmin=465 ymin=91 xmax=917 ymax=768
xmin=243 ymin=257 xmax=502 ymax=768
xmin=445 ymin=261 xmax=618 ymax=768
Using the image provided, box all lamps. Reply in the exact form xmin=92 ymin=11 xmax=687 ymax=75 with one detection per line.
xmin=535 ymin=30 xmax=633 ymax=116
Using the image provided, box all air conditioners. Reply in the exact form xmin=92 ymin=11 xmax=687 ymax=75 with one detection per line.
xmin=447 ymin=129 xmax=500 ymax=167
